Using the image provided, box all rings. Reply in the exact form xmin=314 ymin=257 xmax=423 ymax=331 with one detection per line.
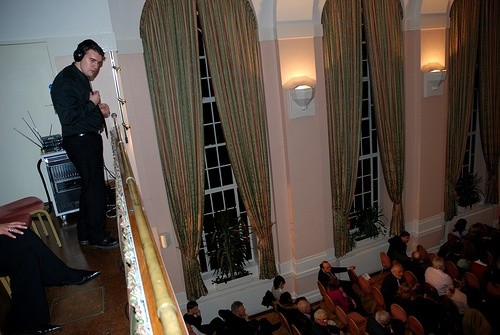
xmin=8 ymin=228 xmax=14 ymax=232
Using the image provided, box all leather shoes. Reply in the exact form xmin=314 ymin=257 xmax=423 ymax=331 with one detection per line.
xmin=49 ymin=269 xmax=100 ymax=285
xmin=78 ymin=230 xmax=120 ymax=249
xmin=32 ymin=325 xmax=61 ymax=335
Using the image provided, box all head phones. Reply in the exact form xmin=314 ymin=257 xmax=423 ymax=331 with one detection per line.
xmin=74 ymin=41 xmax=96 ymax=62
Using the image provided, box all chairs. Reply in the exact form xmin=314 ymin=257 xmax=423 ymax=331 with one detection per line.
xmin=186 ymin=222 xmax=500 ymax=335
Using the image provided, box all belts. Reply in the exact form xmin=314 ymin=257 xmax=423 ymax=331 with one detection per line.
xmin=70 ymin=129 xmax=101 ymax=138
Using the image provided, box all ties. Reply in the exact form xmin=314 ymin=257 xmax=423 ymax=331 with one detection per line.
xmin=88 ymin=81 xmax=109 ymax=139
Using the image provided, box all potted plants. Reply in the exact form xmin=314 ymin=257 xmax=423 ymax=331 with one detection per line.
xmin=197 ymin=204 xmax=258 ymax=291
xmin=348 ymin=202 xmax=387 ymax=247
xmin=452 ymin=172 xmax=486 ymax=212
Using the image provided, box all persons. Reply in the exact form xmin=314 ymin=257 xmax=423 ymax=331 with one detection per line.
xmin=0 ymin=221 xmax=101 ymax=335
xmin=50 ymin=39 xmax=119 ymax=249
xmin=184 ymin=218 xmax=496 ymax=335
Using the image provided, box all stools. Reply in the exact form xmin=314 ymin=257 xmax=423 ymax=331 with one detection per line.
xmin=0 ymin=196 xmax=62 ymax=248
xmin=0 ymin=212 xmax=42 ymax=239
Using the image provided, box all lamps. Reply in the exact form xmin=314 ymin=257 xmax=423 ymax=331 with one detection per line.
xmin=289 ymin=85 xmax=316 ymax=119
xmin=424 ymin=70 xmax=448 ymax=98
